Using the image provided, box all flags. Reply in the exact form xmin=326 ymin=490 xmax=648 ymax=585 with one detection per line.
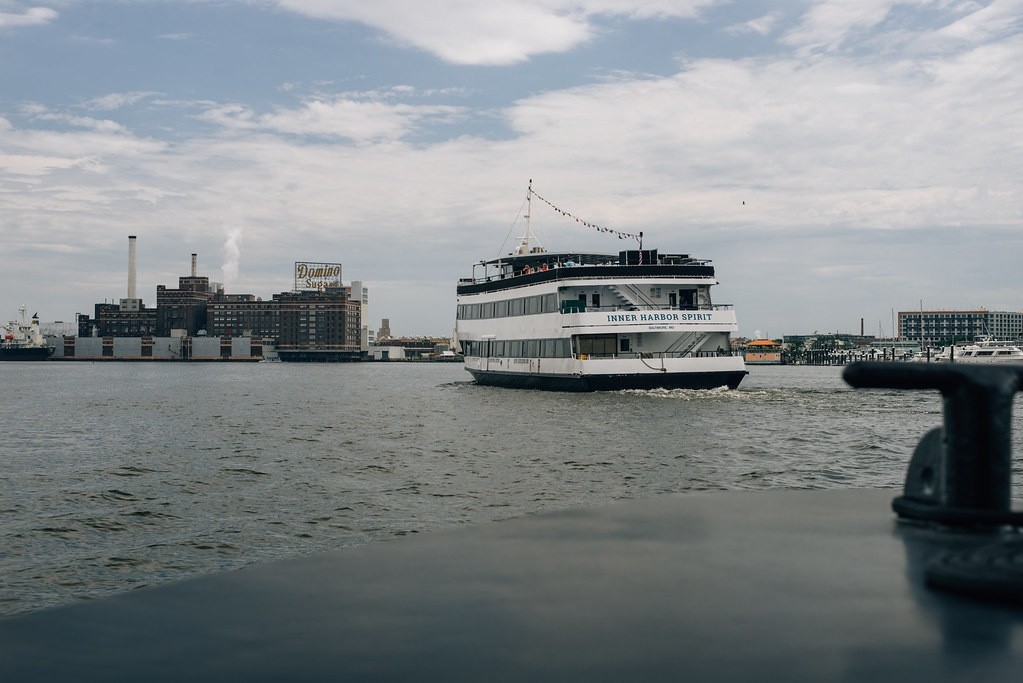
xmin=638 ymin=241 xmax=643 ymax=266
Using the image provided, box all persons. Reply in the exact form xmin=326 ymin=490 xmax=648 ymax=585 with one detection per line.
xmin=541 ymin=263 xmax=549 ymax=271
xmin=717 ymin=346 xmax=721 ymax=357
xmin=524 ymin=264 xmax=531 ymax=274
xmin=487 ymin=277 xmax=491 ymax=282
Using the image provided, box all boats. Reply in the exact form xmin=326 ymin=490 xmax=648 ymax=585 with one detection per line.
xmin=267 ymin=356 xmax=282 ymax=363
xmin=259 ymin=359 xmax=267 ymax=363
xmin=454 ymin=178 xmax=751 ymax=393
xmin=0 ymin=302 xmax=57 ymax=361
xmin=953 ymin=335 xmax=1023 ymax=367
